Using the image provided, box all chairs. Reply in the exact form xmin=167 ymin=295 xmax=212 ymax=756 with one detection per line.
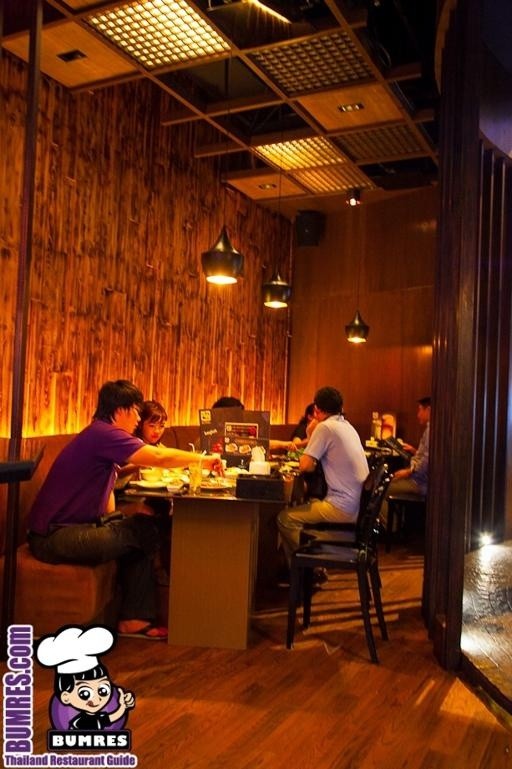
xmin=0 ymin=416 xmax=435 ymax=666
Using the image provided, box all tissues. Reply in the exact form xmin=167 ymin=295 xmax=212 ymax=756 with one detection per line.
xmin=248 ymin=445 xmax=271 ymax=475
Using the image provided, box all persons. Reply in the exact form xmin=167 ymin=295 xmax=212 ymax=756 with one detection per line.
xmin=275 ymin=387 xmax=371 ymax=599
xmin=190 ymin=396 xmax=245 ymax=454
xmin=26 ymin=379 xmax=225 ymax=641
xmin=292 ymin=404 xmax=327 ymax=500
xmin=53 ymin=665 xmax=134 ymax=735
xmin=115 ymin=400 xmax=173 ymax=578
xmin=373 ymin=396 xmax=432 ymax=541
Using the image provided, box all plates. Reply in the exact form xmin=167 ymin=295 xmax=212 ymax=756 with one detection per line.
xmin=130 ymin=480 xmax=166 ymax=490
xmin=226 ymin=443 xmax=238 ymax=453
xmin=239 ymin=445 xmax=252 ymax=455
xmin=166 ymin=458 xmax=245 ymax=496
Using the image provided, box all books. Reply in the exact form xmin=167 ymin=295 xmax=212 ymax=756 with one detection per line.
xmin=384 ymin=435 xmax=414 ymax=462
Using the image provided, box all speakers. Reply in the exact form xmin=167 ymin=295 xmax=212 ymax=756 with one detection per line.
xmin=296 ymin=210 xmax=323 ymax=247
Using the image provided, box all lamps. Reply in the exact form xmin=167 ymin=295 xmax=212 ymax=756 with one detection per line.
xmin=197 ymin=55 xmax=248 ymax=285
xmin=260 ymin=89 xmax=292 ymax=309
xmin=343 ymin=185 xmax=371 ymax=345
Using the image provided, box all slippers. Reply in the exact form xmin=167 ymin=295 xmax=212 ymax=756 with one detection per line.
xmin=117 ymin=622 xmax=168 ymax=640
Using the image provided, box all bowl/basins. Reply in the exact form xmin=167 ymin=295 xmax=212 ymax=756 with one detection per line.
xmin=141 ymin=468 xmax=162 ymax=482
